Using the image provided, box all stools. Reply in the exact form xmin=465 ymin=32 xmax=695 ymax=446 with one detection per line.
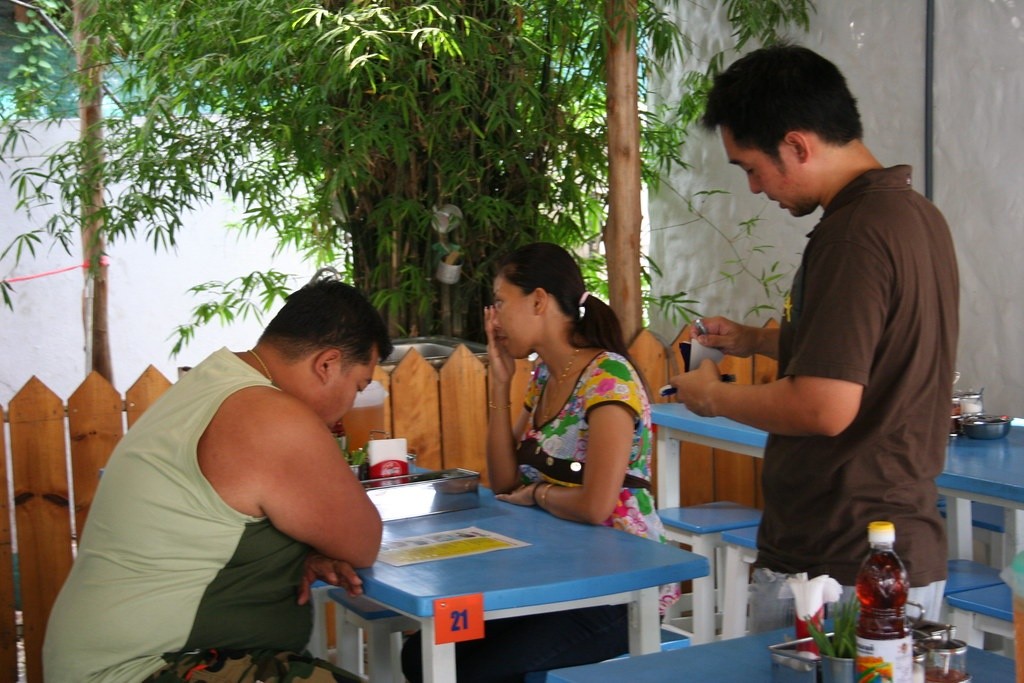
xmin=939 ymin=501 xmax=1014 ymax=649
xmin=657 ymin=500 xmax=762 ymax=644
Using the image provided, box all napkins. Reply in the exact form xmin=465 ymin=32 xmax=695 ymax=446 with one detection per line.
xmin=776 ymin=570 xmax=844 ymax=623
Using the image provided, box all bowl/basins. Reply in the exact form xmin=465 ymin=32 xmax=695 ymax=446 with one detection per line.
xmin=958 ymin=415 xmax=1014 ymax=440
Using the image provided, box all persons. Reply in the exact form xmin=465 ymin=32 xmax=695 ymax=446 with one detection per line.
xmin=669 ymin=44 xmax=958 ymax=634
xmin=400 ymin=243 xmax=682 ymax=683
xmin=43 ymin=281 xmax=396 ymax=682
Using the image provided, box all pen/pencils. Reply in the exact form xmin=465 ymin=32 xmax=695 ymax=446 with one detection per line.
xmin=694 ymin=319 xmax=709 ymax=336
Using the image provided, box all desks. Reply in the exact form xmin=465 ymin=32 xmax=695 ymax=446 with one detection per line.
xmin=643 ymin=403 xmax=1024 ymax=649
xmin=307 ymin=462 xmax=711 ymax=683
xmin=546 ymin=609 xmax=1024 ymax=683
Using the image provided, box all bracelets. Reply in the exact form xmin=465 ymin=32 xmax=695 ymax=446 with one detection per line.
xmin=489 ymin=402 xmax=512 ymax=409
xmin=533 ymin=480 xmax=546 ymax=505
xmin=542 ymin=483 xmax=555 ymax=507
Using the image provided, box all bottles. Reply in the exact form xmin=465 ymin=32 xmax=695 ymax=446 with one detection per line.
xmin=327 ymin=418 xmax=347 ymax=452
xmin=407 ymin=453 xmax=416 ymax=474
xmin=854 ymin=521 xmax=913 ymax=683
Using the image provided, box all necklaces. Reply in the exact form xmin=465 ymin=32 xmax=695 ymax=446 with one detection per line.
xmin=247 ymin=349 xmax=274 ymax=387
xmin=539 ymin=346 xmax=579 ymax=423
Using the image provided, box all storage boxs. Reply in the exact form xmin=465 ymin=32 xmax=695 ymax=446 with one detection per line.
xmin=768 ymin=616 xmax=957 ymax=683
